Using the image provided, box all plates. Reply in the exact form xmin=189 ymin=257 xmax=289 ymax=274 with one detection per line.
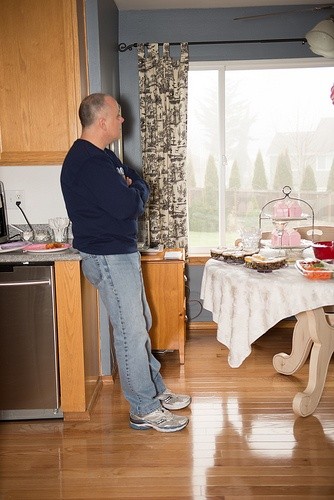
xmin=0 ymin=242 xmax=29 ymax=253
xmin=23 ymin=243 xmax=70 ymax=252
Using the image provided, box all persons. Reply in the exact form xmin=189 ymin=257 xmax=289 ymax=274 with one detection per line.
xmin=60 ymin=93 xmax=192 ymax=433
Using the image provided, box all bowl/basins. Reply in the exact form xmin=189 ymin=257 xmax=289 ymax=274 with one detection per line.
xmin=312 ymin=240 xmax=334 ymax=259
xmin=295 ymin=258 xmax=334 ymax=279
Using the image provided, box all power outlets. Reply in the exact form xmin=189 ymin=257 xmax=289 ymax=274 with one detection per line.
xmin=5 ymin=190 xmax=24 ymax=209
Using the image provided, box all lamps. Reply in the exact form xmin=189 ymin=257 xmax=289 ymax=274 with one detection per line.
xmin=305 ymin=16 xmax=334 ymax=59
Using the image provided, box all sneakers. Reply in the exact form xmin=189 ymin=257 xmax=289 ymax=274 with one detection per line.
xmin=129 ymin=406 xmax=189 ymax=433
xmin=157 ymin=388 xmax=191 ymax=410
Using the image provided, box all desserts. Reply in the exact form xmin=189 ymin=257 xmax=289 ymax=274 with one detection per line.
xmin=210 ymin=248 xmax=286 ymax=270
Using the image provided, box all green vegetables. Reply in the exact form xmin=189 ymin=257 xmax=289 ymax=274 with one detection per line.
xmin=314 ymin=264 xmax=324 ymax=267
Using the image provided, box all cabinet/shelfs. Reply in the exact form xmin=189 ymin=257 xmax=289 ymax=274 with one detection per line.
xmin=0 ymin=0 xmax=90 ymax=166
xmin=199 ymin=257 xmax=334 ymax=417
xmin=141 ymin=261 xmax=188 ymax=366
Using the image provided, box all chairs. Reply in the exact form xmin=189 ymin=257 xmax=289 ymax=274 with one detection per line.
xmin=262 ymin=225 xmax=334 ymax=314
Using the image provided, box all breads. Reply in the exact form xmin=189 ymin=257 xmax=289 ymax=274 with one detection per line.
xmin=46 ymin=242 xmax=61 ymax=249
